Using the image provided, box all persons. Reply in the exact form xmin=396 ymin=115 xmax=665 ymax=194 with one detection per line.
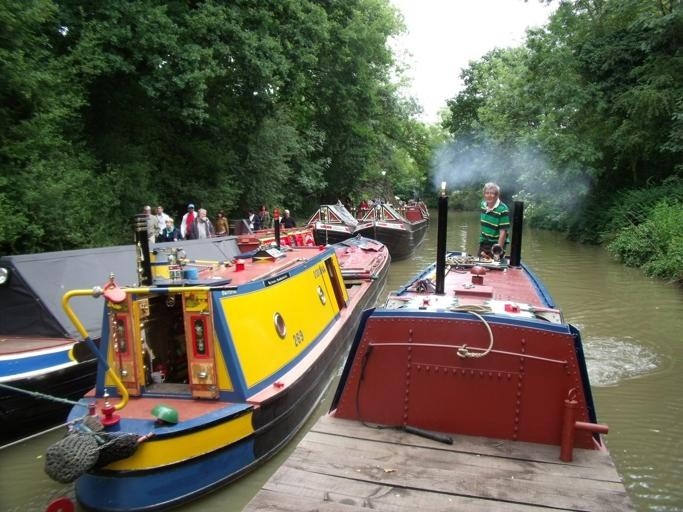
xmin=156 ymin=217 xmax=180 ymax=242
xmin=153 ymin=205 xmax=172 ymax=242
xmin=188 ymin=207 xmax=214 ymax=240
xmin=271 ymin=208 xmax=283 ymax=229
xmin=359 ymin=200 xmax=369 ymax=216
xmin=477 ymin=181 xmax=511 ymax=257
xmin=345 ymin=197 xmax=354 ymax=211
xmin=280 ymin=209 xmax=296 ymax=229
xmin=179 ymin=204 xmax=198 ymax=239
xmin=258 ymin=204 xmax=271 ymax=229
xmin=241 ymin=206 xmax=261 ymax=232
xmin=213 ymin=210 xmax=229 ymax=238
xmin=134 ymin=204 xmax=160 ymax=245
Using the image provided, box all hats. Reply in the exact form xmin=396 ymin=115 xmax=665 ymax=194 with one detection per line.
xmin=187 ymin=203 xmax=194 ymax=209
